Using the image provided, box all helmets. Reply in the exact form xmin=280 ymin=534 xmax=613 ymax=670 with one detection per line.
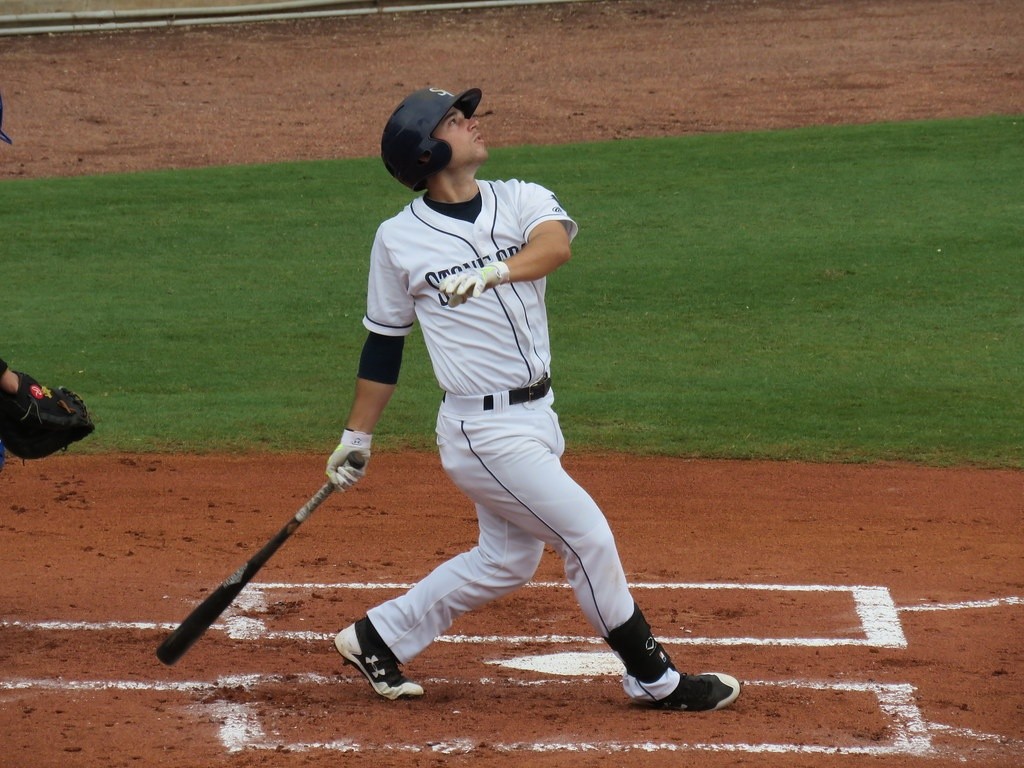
xmin=379 ymin=88 xmax=483 ymax=192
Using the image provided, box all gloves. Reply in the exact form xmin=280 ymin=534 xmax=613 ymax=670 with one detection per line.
xmin=324 ymin=427 xmax=371 ymax=493
xmin=439 ymin=261 xmax=511 ymax=307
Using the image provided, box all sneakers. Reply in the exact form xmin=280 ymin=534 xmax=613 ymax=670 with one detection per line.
xmin=334 ymin=615 xmax=426 ymax=700
xmin=645 ymin=672 xmax=741 ymax=711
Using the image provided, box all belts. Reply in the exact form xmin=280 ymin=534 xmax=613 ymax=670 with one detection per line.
xmin=443 ymin=372 xmax=552 ymax=410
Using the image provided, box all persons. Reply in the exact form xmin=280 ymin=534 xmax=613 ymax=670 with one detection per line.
xmin=0 ymin=359 xmax=93 ymax=461
xmin=324 ymin=85 xmax=740 ymax=714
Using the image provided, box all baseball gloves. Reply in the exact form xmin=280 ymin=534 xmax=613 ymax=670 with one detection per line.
xmin=0 ymin=371 xmax=96 ymax=461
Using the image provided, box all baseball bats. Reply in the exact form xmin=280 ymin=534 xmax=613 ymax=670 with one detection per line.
xmin=156 ymin=451 xmax=364 ymax=666
